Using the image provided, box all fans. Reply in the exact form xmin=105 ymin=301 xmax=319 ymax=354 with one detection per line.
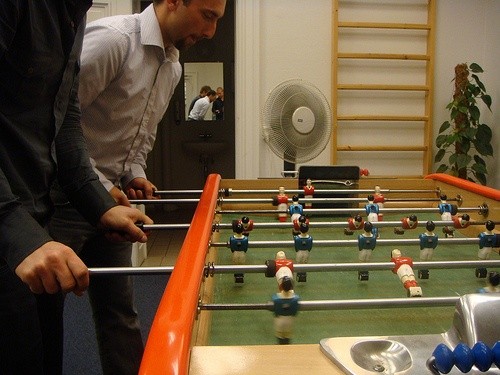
xmin=260 ymin=78 xmax=335 ymax=179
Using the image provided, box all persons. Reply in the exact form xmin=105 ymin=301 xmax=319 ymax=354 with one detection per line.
xmin=79 ymin=0 xmax=227 ymax=375
xmin=187 ymin=85 xmax=224 ymax=120
xmin=0 ymin=0 xmax=155 ymax=375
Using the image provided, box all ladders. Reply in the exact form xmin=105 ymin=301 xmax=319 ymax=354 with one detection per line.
xmin=328 ymin=1 xmax=437 ymax=172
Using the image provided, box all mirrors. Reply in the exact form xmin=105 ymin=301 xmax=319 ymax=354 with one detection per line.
xmin=183 ymin=61 xmax=224 ymax=122
